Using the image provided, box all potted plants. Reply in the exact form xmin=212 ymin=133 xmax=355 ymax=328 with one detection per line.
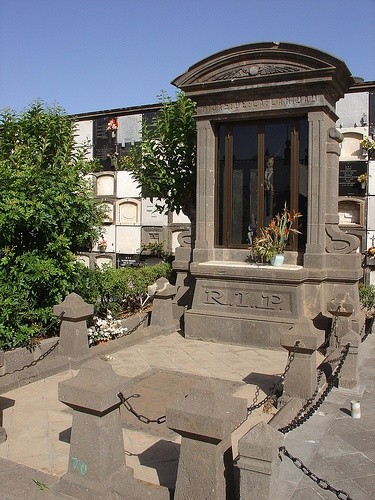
xmin=98 ymin=241 xmax=107 ymax=253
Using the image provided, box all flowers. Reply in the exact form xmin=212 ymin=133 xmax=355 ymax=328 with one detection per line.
xmin=248 ymin=200 xmax=303 ymax=264
xmin=107 ymin=118 xmax=118 ymax=137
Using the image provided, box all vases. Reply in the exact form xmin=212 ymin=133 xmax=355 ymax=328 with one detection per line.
xmin=269 ymin=254 xmax=284 ymax=266
